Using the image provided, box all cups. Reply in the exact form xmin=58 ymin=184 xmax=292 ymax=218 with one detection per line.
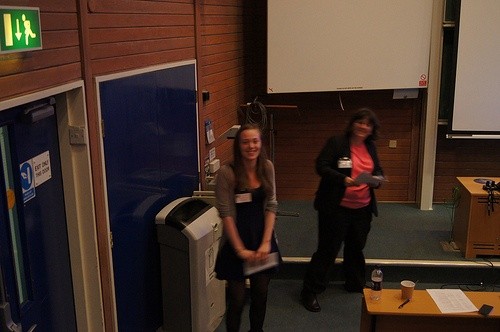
xmin=401 ymin=280 xmax=415 ymax=300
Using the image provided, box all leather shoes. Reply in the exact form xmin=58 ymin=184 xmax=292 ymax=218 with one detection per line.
xmin=301 ymin=288 xmax=321 ymax=312
xmin=359 ymin=281 xmax=373 ymax=293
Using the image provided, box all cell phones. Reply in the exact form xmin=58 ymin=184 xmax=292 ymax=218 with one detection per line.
xmin=479 ymin=304 xmax=494 ymax=315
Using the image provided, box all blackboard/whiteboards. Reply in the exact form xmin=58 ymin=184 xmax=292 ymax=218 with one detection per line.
xmin=267 ymin=0 xmax=434 ymax=93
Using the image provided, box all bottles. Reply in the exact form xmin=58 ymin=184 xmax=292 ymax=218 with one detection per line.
xmin=370 ymin=265 xmax=383 ymax=301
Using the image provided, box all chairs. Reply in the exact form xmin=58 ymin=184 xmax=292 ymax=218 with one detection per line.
xmin=440 ymin=271 xmax=489 ymax=292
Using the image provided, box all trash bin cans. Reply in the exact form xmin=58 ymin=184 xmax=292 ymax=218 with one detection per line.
xmin=155 ymin=195 xmax=224 ymax=331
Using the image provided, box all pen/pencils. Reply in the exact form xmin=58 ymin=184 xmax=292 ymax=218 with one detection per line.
xmin=399 ymin=299 xmax=410 ymax=309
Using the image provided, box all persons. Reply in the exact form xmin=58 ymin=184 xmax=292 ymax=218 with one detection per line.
xmin=298 ymin=107 xmax=383 ymax=311
xmin=213 ymin=122 xmax=284 ymax=332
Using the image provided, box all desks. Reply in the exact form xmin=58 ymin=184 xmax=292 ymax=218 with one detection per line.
xmin=360 ymin=288 xmax=500 ymax=332
xmin=452 ymin=176 xmax=500 ymax=259
